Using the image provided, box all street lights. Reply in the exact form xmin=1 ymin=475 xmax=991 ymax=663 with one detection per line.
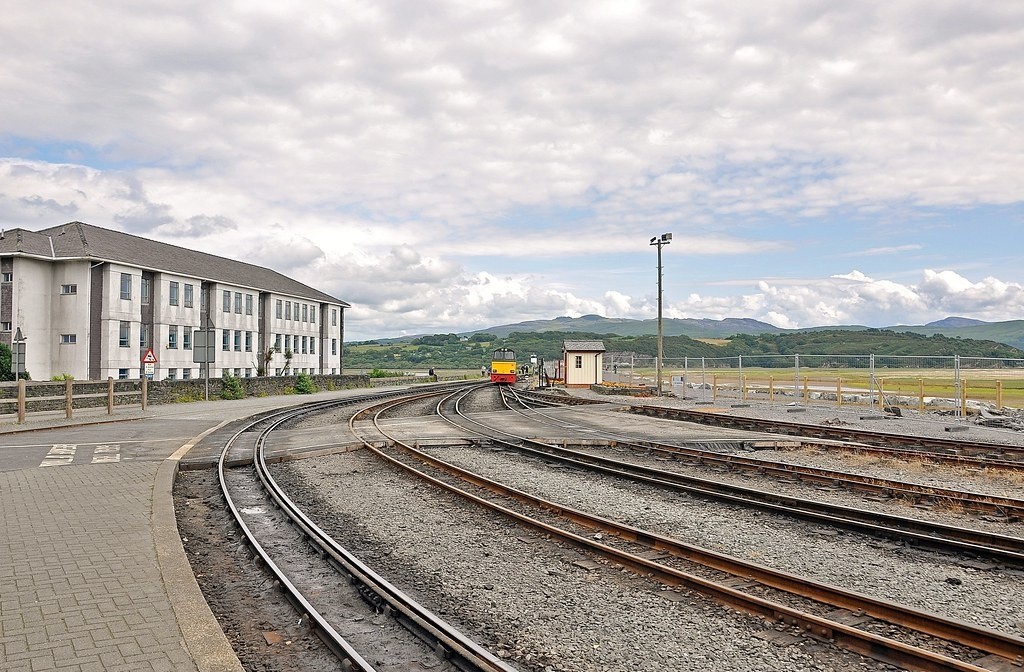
xmin=650 ymin=233 xmax=674 ymax=396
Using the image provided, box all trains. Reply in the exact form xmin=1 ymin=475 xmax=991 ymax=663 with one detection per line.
xmin=530 ymin=354 xmax=538 ymax=364
xmin=490 ymin=346 xmax=518 ymax=384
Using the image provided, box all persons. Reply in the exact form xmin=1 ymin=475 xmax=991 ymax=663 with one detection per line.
xmin=481 ymin=361 xmax=492 ymax=377
xmin=521 ymin=364 xmax=529 ymax=374
xmin=429 ymin=366 xmax=439 ymax=382
